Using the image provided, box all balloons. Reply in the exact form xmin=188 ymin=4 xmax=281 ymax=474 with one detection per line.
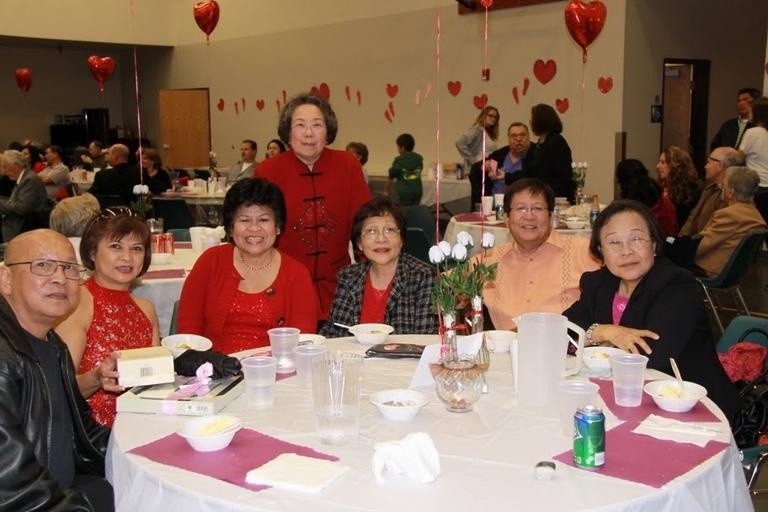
xmin=18 ymin=68 xmax=33 ymax=94
xmin=89 ymin=55 xmax=116 ymax=81
xmin=190 ymin=0 xmax=228 ymax=39
xmin=562 ymin=0 xmax=612 ymax=49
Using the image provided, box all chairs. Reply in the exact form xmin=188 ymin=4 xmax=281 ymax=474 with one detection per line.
xmin=693 ymin=226 xmax=768 ymax=496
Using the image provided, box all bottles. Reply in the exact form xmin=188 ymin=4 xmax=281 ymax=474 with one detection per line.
xmin=174 ymin=178 xmax=180 ymax=192
xmin=455 ymin=161 xmax=462 ymax=179
xmin=81 ymin=169 xmax=87 ymax=182
xmin=588 ymin=195 xmax=600 ymax=229
xmin=551 ymin=196 xmax=569 ymax=229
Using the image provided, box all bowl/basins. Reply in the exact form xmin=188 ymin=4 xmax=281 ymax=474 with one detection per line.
xmin=576 ymin=347 xmax=626 ymax=376
xmin=347 ymin=323 xmax=394 ymax=347
xmin=175 ymin=414 xmax=243 ymax=452
xmin=643 ymin=380 xmax=708 ymax=413
xmin=368 ymin=390 xmax=429 ymax=421
xmin=482 ymin=329 xmax=514 ymax=353
xmin=563 ymin=217 xmax=586 ymax=229
xmin=240 ymin=356 xmax=277 ymax=387
xmin=160 ymin=333 xmax=213 ymax=359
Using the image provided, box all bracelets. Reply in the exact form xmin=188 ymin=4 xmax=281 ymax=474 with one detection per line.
xmin=587 ymin=321 xmax=602 ymax=347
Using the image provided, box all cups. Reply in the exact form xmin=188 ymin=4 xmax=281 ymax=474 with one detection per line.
xmin=292 ymin=345 xmax=326 ymax=386
xmin=559 ymin=380 xmax=600 ymax=436
xmin=608 ymin=353 xmax=648 ymax=408
xmin=494 ymin=194 xmax=503 ymax=207
xmin=267 ymin=327 xmax=301 ymax=374
xmin=309 ymin=353 xmax=365 ymax=443
xmin=481 ymin=196 xmax=493 ymax=216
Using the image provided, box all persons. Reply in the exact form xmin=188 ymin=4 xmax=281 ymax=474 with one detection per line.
xmin=252 ymin=94 xmax=377 ymax=333
xmin=456 ymin=103 xmax=577 ymax=205
xmin=0 ymin=140 xmax=172 ymax=245
xmin=389 ymin=134 xmax=424 ymax=205
xmin=0 ymin=225 xmax=117 ymax=507
xmin=616 ymin=144 xmax=766 ymax=281
xmin=169 ymin=178 xmax=318 ymax=357
xmin=54 ymin=210 xmax=160 ymax=428
xmin=710 ymin=88 xmax=768 ymax=219
xmin=320 ymin=200 xmax=442 ymax=339
xmin=440 ymin=179 xmax=607 ymax=332
xmin=347 ymin=142 xmax=370 ymax=184
xmin=229 ymin=138 xmax=286 ymax=183
xmin=560 ymin=199 xmax=716 ymax=406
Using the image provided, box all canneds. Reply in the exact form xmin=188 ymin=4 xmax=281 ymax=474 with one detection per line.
xmin=574 ymin=406 xmax=604 ymax=470
xmin=590 ymin=211 xmax=599 ymax=226
xmin=152 ymin=234 xmax=165 ymax=253
xmin=552 ymin=209 xmax=560 ymax=227
xmin=164 ymin=232 xmax=175 ymax=254
xmin=496 ymin=204 xmax=503 ymax=220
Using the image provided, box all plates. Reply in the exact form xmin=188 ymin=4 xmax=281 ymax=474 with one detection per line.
xmin=297 ymin=334 xmax=326 ymax=347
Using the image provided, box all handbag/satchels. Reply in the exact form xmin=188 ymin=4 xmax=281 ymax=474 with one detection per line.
xmin=365 ymin=343 xmax=425 ymax=358
xmin=174 ymin=350 xmax=242 ymax=380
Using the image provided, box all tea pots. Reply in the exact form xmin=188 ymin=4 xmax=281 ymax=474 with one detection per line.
xmin=512 ymin=311 xmax=585 ymax=395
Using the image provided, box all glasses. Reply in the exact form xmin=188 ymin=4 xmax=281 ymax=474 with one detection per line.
xmin=707 ymin=157 xmax=720 ymax=162
xmin=600 ymin=237 xmax=653 ymax=250
xmin=488 ymin=115 xmax=498 ymax=119
xmin=507 ymin=206 xmax=547 ymax=215
xmin=719 ymin=183 xmax=731 ymax=192
xmin=6 ymin=258 xmax=88 ymax=281
xmin=95 ymin=206 xmax=144 ymax=219
xmin=360 ymin=224 xmax=400 ymax=238
xmin=509 ymin=134 xmax=525 ymax=138
